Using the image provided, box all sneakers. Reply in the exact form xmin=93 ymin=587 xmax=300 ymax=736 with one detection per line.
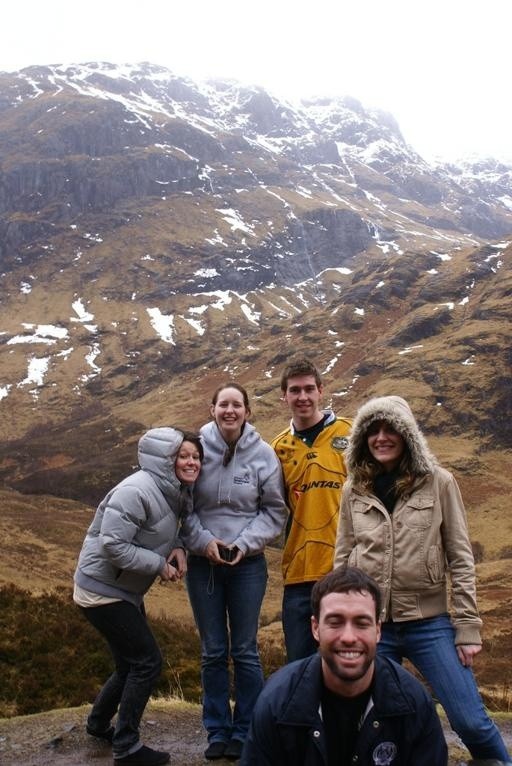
xmin=87 ymin=726 xmax=115 ymax=744
xmin=205 ymin=740 xmax=243 ymax=759
xmin=113 ymin=745 xmax=170 ymax=766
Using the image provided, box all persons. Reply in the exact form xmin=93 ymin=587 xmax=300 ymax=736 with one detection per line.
xmin=331 ymin=396 xmax=511 ymax=765
xmin=71 ymin=425 xmax=205 ymax=766
xmin=177 ymin=382 xmax=289 ymax=761
xmin=235 ymin=563 xmax=451 ymax=766
xmin=270 ymin=355 xmax=353 ymax=663
